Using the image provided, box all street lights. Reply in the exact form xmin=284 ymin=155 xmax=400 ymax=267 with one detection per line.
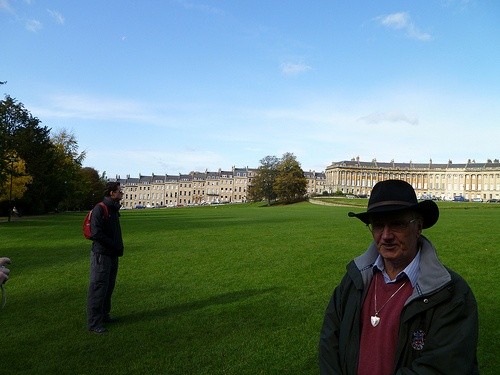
xmin=8 ymin=155 xmax=15 ymax=222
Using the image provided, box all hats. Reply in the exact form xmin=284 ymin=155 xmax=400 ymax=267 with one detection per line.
xmin=348 ymin=179 xmax=439 ymax=229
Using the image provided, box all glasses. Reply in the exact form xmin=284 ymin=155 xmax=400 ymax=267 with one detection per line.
xmin=366 ymin=219 xmax=416 ymax=234
xmin=115 ymin=190 xmax=122 ymax=194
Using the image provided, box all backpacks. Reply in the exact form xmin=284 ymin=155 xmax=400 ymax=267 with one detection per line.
xmin=83 ymin=201 xmax=109 ymax=239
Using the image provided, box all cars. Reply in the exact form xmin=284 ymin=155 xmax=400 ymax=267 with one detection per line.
xmin=120 ymin=206 xmax=128 ymax=210
xmin=135 ymin=205 xmax=146 ymax=208
xmin=471 ymin=197 xmax=483 ymax=202
xmin=198 ymin=201 xmax=210 ymax=206
xmin=451 ymin=195 xmax=469 ymax=202
xmin=417 ymin=193 xmax=432 ymax=200
xmin=177 ymin=203 xmax=184 ymax=207
xmin=486 ymin=198 xmax=500 ymax=203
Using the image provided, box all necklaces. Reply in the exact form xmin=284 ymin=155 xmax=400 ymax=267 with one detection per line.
xmin=370 ymin=272 xmax=407 ymax=328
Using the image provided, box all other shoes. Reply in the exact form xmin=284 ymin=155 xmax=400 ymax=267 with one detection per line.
xmin=93 ymin=326 xmax=107 ymax=334
xmin=102 ymin=314 xmax=115 ymax=323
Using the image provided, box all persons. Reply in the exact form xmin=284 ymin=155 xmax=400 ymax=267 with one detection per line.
xmin=0 ymin=257 xmax=11 ymax=286
xmin=318 ymin=180 xmax=480 ymax=374
xmin=86 ymin=182 xmax=124 ymax=333
